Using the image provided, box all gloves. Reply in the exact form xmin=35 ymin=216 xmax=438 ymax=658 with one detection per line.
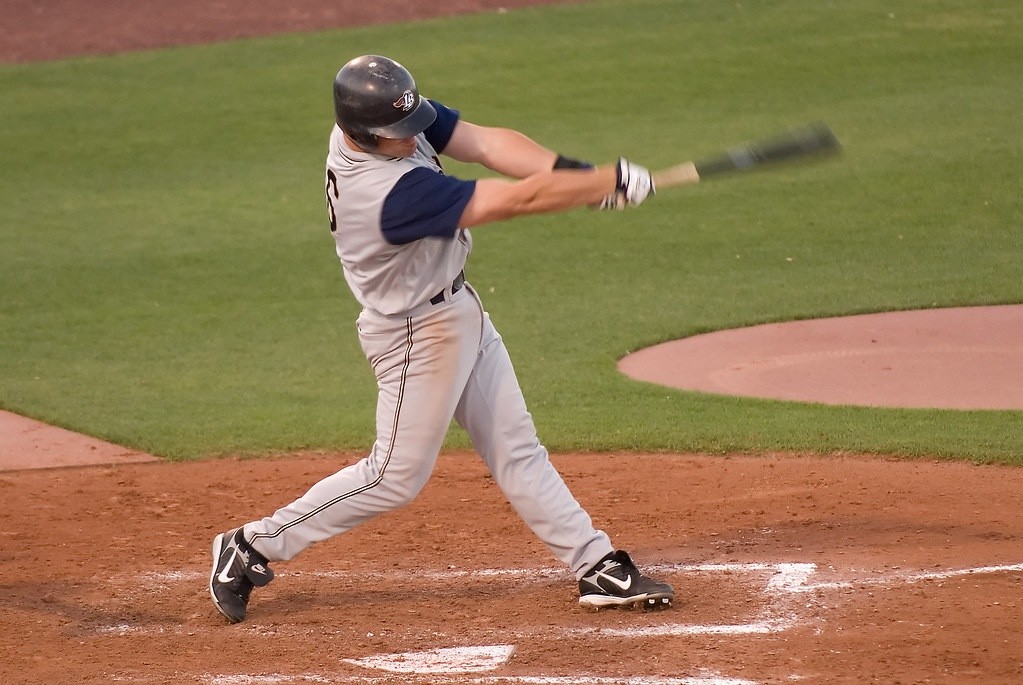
xmin=598 ymin=153 xmax=657 ymax=210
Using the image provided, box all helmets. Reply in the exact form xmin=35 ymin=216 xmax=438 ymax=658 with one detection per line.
xmin=333 ymin=55 xmax=438 ymax=147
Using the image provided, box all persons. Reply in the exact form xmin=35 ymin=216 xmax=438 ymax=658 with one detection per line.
xmin=210 ymin=55 xmax=676 ymax=623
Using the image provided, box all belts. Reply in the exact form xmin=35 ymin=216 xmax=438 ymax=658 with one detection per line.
xmin=430 ymin=268 xmax=465 ymax=305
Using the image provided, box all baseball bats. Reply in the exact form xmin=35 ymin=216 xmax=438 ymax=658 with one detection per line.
xmin=587 ymin=121 xmax=845 ymax=214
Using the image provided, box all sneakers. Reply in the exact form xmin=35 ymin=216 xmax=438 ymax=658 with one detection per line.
xmin=209 ymin=525 xmax=274 ymax=623
xmin=579 ymin=550 xmax=675 ymax=611
